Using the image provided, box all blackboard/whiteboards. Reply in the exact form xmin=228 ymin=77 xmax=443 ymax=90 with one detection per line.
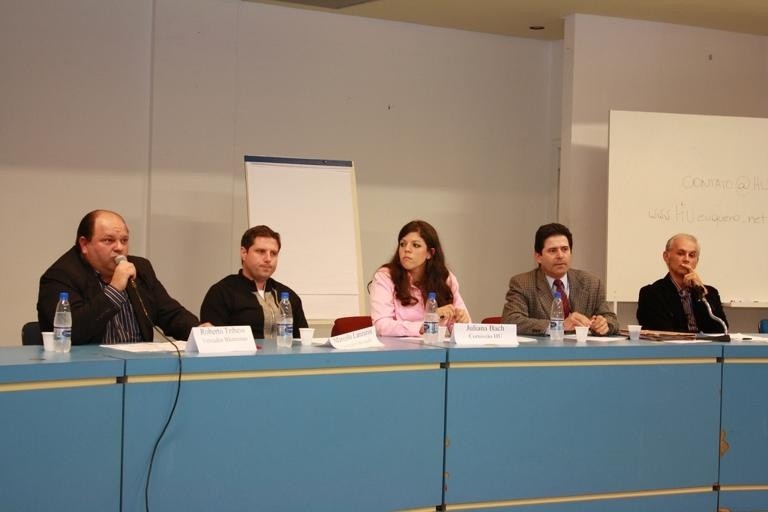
xmin=244 ymin=155 xmax=364 ymax=320
xmin=605 ymin=109 xmax=768 ymax=308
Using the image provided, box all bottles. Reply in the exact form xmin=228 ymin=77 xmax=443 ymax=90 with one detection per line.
xmin=548 ymin=292 xmax=565 ymax=341
xmin=274 ymin=290 xmax=294 ymax=347
xmin=54 ymin=292 xmax=74 ymax=354
xmin=423 ymin=293 xmax=439 ymax=345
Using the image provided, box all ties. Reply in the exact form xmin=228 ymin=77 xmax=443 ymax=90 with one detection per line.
xmin=680 ymin=289 xmax=699 ymax=333
xmin=552 ymin=279 xmax=570 ymax=321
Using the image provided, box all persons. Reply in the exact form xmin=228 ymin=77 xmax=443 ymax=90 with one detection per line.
xmin=636 ymin=232 xmax=729 ymax=334
xmin=36 ymin=209 xmax=213 ymax=344
xmin=200 ymin=225 xmax=312 ymax=340
xmin=502 ymin=224 xmax=620 ymax=337
xmin=366 ymin=219 xmax=473 ymax=336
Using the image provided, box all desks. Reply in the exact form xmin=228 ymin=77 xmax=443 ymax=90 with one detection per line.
xmin=393 ymin=329 xmax=723 ymax=511
xmin=666 ymin=330 xmax=768 ymax=512
xmin=69 ymin=335 xmax=449 ymax=511
xmin=0 ymin=343 xmax=126 ymax=512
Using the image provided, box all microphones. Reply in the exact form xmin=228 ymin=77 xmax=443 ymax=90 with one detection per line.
xmin=694 ymin=284 xmax=732 ymax=342
xmin=113 ymin=256 xmax=137 ymax=287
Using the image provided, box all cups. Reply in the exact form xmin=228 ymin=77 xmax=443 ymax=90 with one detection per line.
xmin=41 ymin=331 xmax=55 ymax=351
xmin=573 ymin=327 xmax=589 ymax=343
xmin=627 ymin=323 xmax=642 ymax=341
xmin=436 ymin=326 xmax=448 ymax=342
xmin=299 ymin=328 xmax=314 ymax=346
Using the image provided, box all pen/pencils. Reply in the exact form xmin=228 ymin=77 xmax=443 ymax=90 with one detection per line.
xmin=743 ymin=338 xmax=752 ymax=340
xmin=256 ymin=344 xmax=262 ymax=349
xmin=440 ymin=315 xmax=444 ymax=319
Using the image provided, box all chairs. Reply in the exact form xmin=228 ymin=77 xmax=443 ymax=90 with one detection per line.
xmin=481 ymin=316 xmax=502 ymax=325
xmin=21 ymin=323 xmax=43 ymax=347
xmin=330 ymin=316 xmax=371 ymax=338
